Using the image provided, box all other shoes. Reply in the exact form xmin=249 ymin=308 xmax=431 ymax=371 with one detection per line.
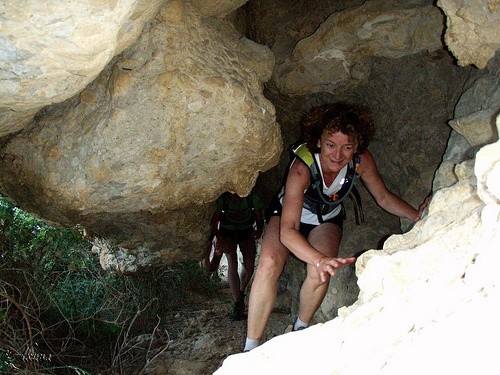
xmin=230 ymin=292 xmax=245 ymax=320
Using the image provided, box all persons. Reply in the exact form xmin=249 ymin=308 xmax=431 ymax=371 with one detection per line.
xmin=243 ymin=101 xmax=433 ymax=352
xmin=204 ymin=214 xmax=223 ymax=283
xmin=211 ymin=187 xmax=264 ymax=320
